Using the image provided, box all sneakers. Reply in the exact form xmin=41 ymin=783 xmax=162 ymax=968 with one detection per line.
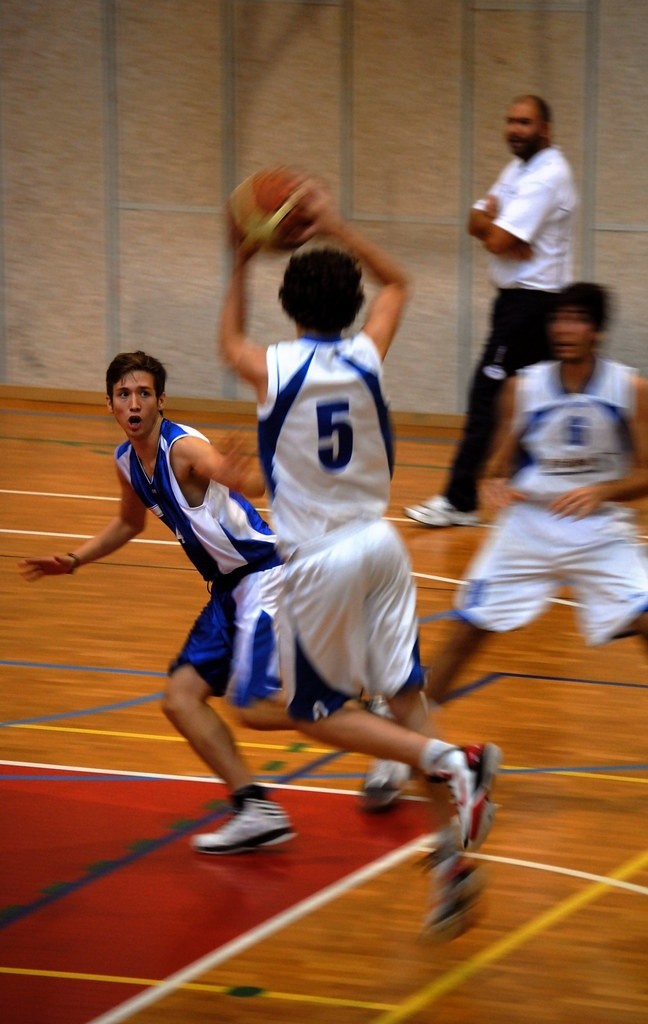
xmin=189 ymin=797 xmax=298 ymax=855
xmin=427 ymin=730 xmax=502 ymax=852
xmin=416 ymin=852 xmax=491 ymax=947
xmin=358 ymin=750 xmax=413 ymax=814
xmin=402 ymin=494 xmax=483 ymax=528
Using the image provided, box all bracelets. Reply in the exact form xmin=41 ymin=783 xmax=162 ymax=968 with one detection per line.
xmin=65 ymin=553 xmax=79 ymax=565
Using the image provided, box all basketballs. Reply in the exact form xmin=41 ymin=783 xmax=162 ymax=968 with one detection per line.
xmin=226 ymin=166 xmax=320 ymax=252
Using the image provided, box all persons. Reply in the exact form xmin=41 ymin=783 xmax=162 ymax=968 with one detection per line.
xmin=363 ymin=281 xmax=647 ymax=809
xmin=405 ymin=94 xmax=603 ymax=527
xmin=18 ymin=353 xmax=396 ymax=855
xmin=217 ymin=178 xmax=499 ymax=938
